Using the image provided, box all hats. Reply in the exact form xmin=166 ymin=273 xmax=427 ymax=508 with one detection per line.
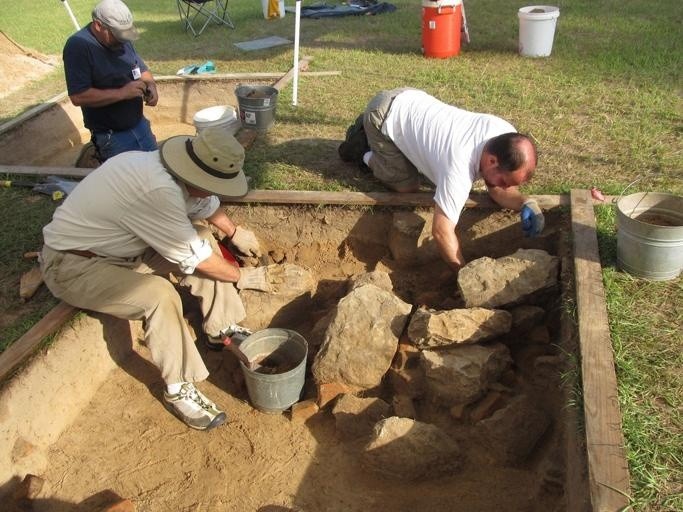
xmin=92 ymin=0 xmax=140 ymax=42
xmin=161 ymin=129 xmax=249 ymax=199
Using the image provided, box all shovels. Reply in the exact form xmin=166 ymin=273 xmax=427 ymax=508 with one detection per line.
xmin=0 ymin=174 xmax=80 ymax=199
xmin=220 ymin=330 xmax=277 ymax=374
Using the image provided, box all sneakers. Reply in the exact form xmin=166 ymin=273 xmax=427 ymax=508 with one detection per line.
xmin=162 ymin=382 xmax=226 ymax=430
xmin=75 ymin=142 xmax=100 ymax=167
xmin=338 ymin=115 xmax=371 ymax=168
xmin=206 ymin=325 xmax=253 ymax=350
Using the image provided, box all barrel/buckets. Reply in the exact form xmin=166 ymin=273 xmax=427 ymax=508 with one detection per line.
xmin=517 ymin=5 xmax=560 ymax=57
xmin=192 ymin=105 xmax=239 ymax=136
xmin=612 ymin=172 xmax=683 ymax=281
xmin=238 ymin=327 xmax=309 ymax=414
xmin=420 ymin=0 xmax=466 ymax=59
xmin=234 ymin=83 xmax=279 ymax=128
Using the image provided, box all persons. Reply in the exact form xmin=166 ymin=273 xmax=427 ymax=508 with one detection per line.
xmin=37 ymin=125 xmax=288 ymax=431
xmin=337 ymin=86 xmax=547 ymax=274
xmin=60 ymin=0 xmax=162 ymax=165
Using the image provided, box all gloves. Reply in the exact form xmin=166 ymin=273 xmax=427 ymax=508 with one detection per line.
xmin=230 ymin=226 xmax=262 ymax=259
xmin=520 ymin=200 xmax=545 ymax=236
xmin=236 ymin=264 xmax=284 ymax=295
